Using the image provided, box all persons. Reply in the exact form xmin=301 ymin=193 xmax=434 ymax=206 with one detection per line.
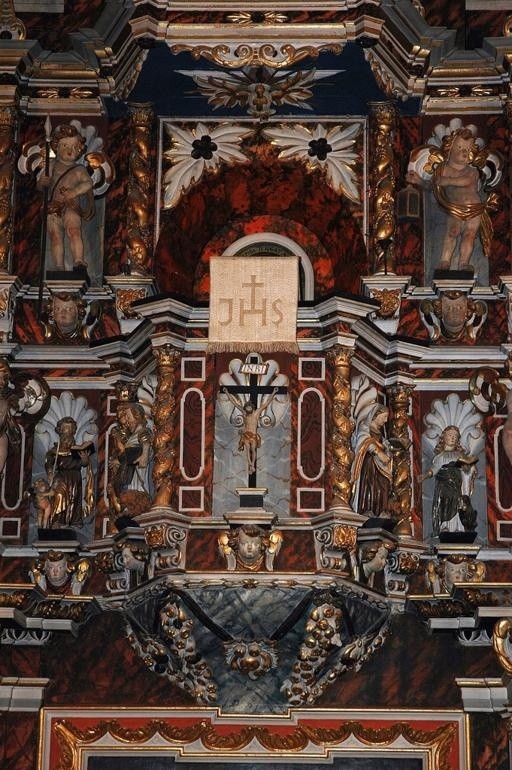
xmin=48 ymin=292 xmax=84 ymax=332
xmin=227 ymin=523 xmax=268 ymax=564
xmin=353 ymin=403 xmax=402 ymax=518
xmin=31 ymin=478 xmax=57 ymax=529
xmin=405 ymin=128 xmax=486 ymax=270
xmin=222 ymin=387 xmax=280 ymax=475
xmin=34 ymin=124 xmax=95 ymax=273
xmin=493 ymin=351 xmax=512 ymax=466
xmin=116 ymin=403 xmax=151 ymax=493
xmin=44 ymin=416 xmax=96 ymax=532
xmin=416 ymin=426 xmax=480 ymax=540
xmin=43 ymin=550 xmax=75 ymax=584
xmin=1 ymin=363 xmax=21 ymax=478
xmin=431 ymin=289 xmax=478 ymax=337
xmin=359 ymin=540 xmax=395 ymax=579
xmin=436 ymin=554 xmax=477 ymax=592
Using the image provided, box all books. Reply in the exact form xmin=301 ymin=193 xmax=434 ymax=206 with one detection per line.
xmin=125 ymin=447 xmax=144 ymax=466
xmin=455 ymin=455 xmax=479 ymax=469
xmin=389 ymin=437 xmax=412 ymax=451
xmin=69 ymin=440 xmax=94 ymax=460
